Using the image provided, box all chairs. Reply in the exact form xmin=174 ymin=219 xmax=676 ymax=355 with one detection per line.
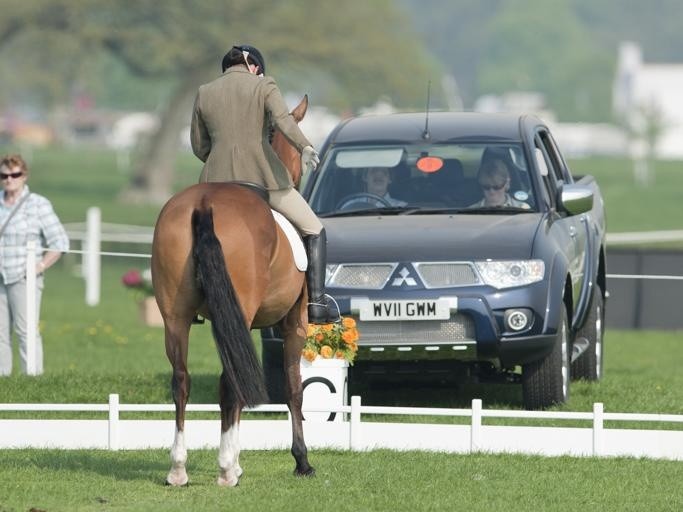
xmin=429 ymin=159 xmax=464 ymax=186
xmin=482 ymin=146 xmax=532 ymax=202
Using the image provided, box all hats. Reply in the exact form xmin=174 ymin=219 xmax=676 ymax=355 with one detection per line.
xmin=223 ymin=44 xmax=265 ymax=75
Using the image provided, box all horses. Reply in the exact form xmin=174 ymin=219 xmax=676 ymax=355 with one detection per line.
xmin=150 ymin=94 xmax=315 ymax=490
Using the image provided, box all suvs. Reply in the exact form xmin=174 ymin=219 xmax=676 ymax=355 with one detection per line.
xmin=259 ymin=80 xmax=607 ymax=405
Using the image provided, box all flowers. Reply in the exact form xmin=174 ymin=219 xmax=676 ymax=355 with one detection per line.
xmin=303 ymin=317 xmax=359 ymax=367
xmin=119 ymin=268 xmax=156 ymax=297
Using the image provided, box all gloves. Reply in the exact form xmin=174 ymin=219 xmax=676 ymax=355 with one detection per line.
xmin=301 ymin=146 xmax=320 ymax=176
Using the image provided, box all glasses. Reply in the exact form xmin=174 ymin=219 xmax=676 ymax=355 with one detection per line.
xmin=1 ymin=172 xmax=22 ymax=179
xmin=480 ymin=183 xmax=505 ymax=191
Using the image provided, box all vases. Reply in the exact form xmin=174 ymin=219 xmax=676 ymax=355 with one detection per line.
xmin=300 ymin=355 xmax=350 ymax=420
xmin=138 ymin=296 xmax=165 ymax=328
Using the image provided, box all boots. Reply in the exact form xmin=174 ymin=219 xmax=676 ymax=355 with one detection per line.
xmin=302 ymin=227 xmax=339 ymax=323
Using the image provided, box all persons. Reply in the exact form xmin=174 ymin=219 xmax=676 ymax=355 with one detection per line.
xmin=191 ymin=45 xmax=341 ymax=325
xmin=339 ymin=167 xmax=410 ymax=211
xmin=0 ymin=154 xmax=71 ymax=377
xmin=466 ymin=157 xmax=531 ymax=210
